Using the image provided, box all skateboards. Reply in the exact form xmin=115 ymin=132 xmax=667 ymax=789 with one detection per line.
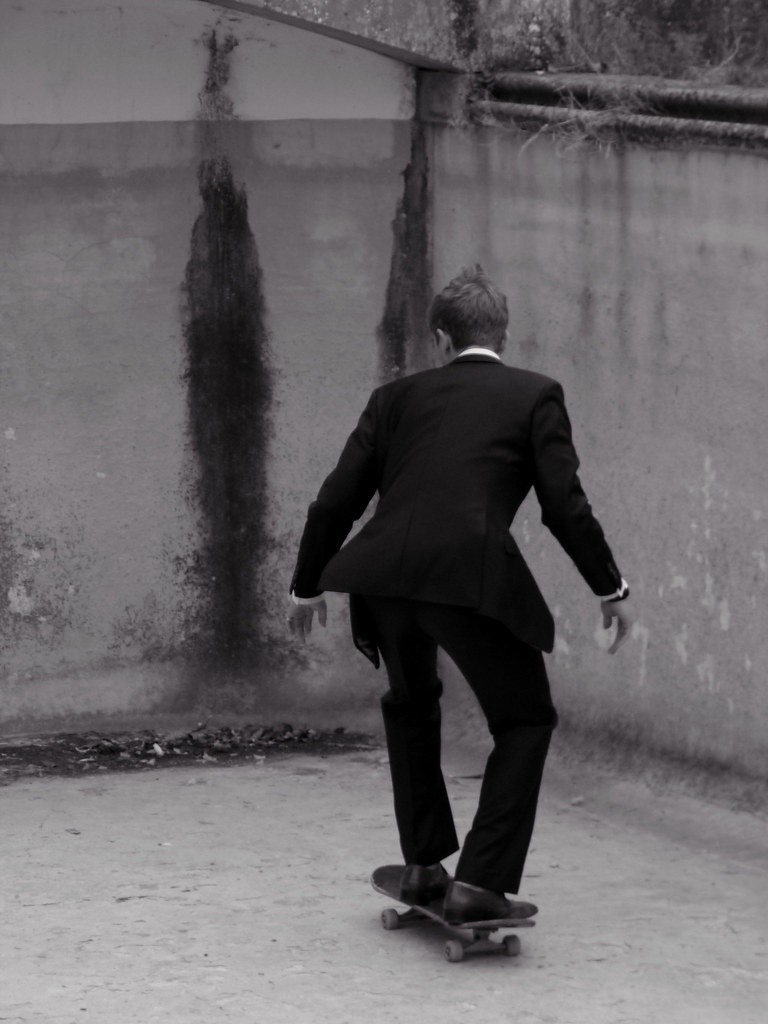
xmin=370 ymin=864 xmax=537 ymax=963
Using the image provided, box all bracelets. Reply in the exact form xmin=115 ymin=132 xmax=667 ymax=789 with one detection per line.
xmin=603 ymin=586 xmax=629 ymax=602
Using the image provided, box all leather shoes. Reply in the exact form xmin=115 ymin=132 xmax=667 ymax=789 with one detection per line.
xmin=400 ymin=863 xmax=452 ymax=906
xmin=443 ymin=883 xmax=538 ymax=925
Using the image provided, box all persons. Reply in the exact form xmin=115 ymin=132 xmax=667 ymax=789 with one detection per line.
xmin=287 ymin=261 xmax=636 ymax=926
